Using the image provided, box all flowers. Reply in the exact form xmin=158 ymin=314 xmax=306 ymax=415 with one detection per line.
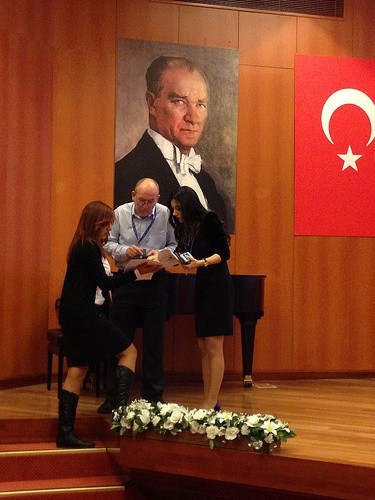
xmin=108 ymin=398 xmax=298 ymax=454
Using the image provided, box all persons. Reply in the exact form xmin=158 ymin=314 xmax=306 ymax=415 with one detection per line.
xmin=96 ymin=178 xmax=179 ymax=414
xmin=114 ymin=56 xmax=230 ymax=234
xmin=55 ymin=201 xmax=163 ymax=448
xmin=167 ymin=185 xmax=233 ymax=410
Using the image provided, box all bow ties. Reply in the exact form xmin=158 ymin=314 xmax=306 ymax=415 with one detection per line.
xmin=179 ymin=153 xmax=202 ymax=176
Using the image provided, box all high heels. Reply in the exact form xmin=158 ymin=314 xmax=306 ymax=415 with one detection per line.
xmin=213 ymin=401 xmax=221 ymax=413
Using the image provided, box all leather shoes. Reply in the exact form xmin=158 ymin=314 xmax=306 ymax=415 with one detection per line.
xmin=139 ymin=386 xmax=166 ymax=406
xmin=97 ymin=397 xmax=115 ymax=413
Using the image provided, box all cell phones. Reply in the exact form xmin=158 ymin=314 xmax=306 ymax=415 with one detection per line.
xmin=180 ymin=252 xmax=195 ymax=264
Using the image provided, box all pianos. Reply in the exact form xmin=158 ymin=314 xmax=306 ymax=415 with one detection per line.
xmin=55 ymin=272 xmax=267 ymax=390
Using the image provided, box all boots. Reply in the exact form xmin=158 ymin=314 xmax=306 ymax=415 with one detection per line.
xmin=112 ymin=364 xmax=135 ymax=408
xmin=56 ymin=389 xmax=96 ymax=449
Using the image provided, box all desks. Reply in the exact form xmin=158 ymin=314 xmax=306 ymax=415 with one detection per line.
xmin=106 ymin=273 xmax=267 ymax=390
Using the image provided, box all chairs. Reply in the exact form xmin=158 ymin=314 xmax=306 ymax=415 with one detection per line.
xmin=44 ymin=297 xmax=102 ymax=398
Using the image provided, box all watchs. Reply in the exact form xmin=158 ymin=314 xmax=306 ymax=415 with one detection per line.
xmin=202 ymin=258 xmax=209 ymax=267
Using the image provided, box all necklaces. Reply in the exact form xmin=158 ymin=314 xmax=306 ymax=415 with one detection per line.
xmin=131 ymin=203 xmax=156 ymax=244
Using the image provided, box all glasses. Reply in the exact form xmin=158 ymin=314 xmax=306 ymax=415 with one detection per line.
xmin=135 ymin=194 xmax=158 ymax=204
